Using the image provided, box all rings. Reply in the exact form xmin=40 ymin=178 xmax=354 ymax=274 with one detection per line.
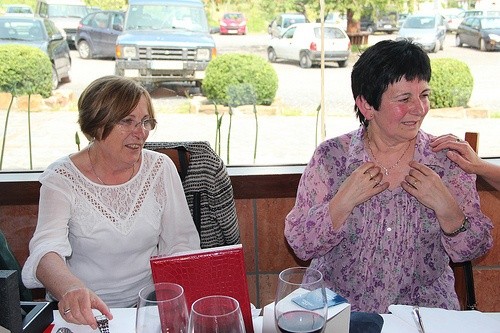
xmin=62 ymin=308 xmax=70 ymax=314
xmin=456 ymin=139 xmax=460 ymax=143
xmin=413 ymin=180 xmax=418 ymax=186
xmin=373 ymin=177 xmax=378 ymax=185
xmin=366 ymin=170 xmax=373 ymax=178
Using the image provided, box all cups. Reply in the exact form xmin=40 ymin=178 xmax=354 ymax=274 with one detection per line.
xmin=274 ymin=266 xmax=328 ymax=333
xmin=135 ymin=282 xmax=190 ymax=333
xmin=189 ymin=296 xmax=248 ymax=333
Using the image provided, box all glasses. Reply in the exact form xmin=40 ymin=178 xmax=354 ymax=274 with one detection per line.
xmin=111 ymin=118 xmax=157 ymax=131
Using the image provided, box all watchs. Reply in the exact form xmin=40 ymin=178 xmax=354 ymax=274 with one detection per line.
xmin=441 ymin=215 xmax=472 ymax=237
xmin=95 ymin=314 xmax=110 ymax=333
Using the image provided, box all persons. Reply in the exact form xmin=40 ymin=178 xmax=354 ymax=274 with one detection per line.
xmin=284 ymin=40 xmax=494 ymax=315
xmin=429 ymin=133 xmax=500 ymax=193
xmin=21 ymin=76 xmax=200 ymax=330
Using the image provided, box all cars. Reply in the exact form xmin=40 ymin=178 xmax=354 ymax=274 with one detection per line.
xmin=443 ymin=7 xmax=500 ymax=51
xmin=219 ymin=11 xmax=248 ymax=36
xmin=265 ymin=12 xmax=351 ymax=69
xmin=0 ymin=0 xmax=126 ymax=90
xmin=396 ymin=10 xmax=446 ymax=52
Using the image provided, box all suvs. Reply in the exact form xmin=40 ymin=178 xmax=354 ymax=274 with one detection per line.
xmin=114 ymin=0 xmax=221 ymax=97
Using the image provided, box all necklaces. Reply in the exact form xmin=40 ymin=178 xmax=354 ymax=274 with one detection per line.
xmin=87 ymin=145 xmax=135 ymax=185
xmin=365 ymin=125 xmax=413 ymax=175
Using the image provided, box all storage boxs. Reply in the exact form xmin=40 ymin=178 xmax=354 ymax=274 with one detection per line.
xmin=262 ymin=287 xmax=351 ymax=333
xmin=0 ymin=269 xmax=54 ymax=333
